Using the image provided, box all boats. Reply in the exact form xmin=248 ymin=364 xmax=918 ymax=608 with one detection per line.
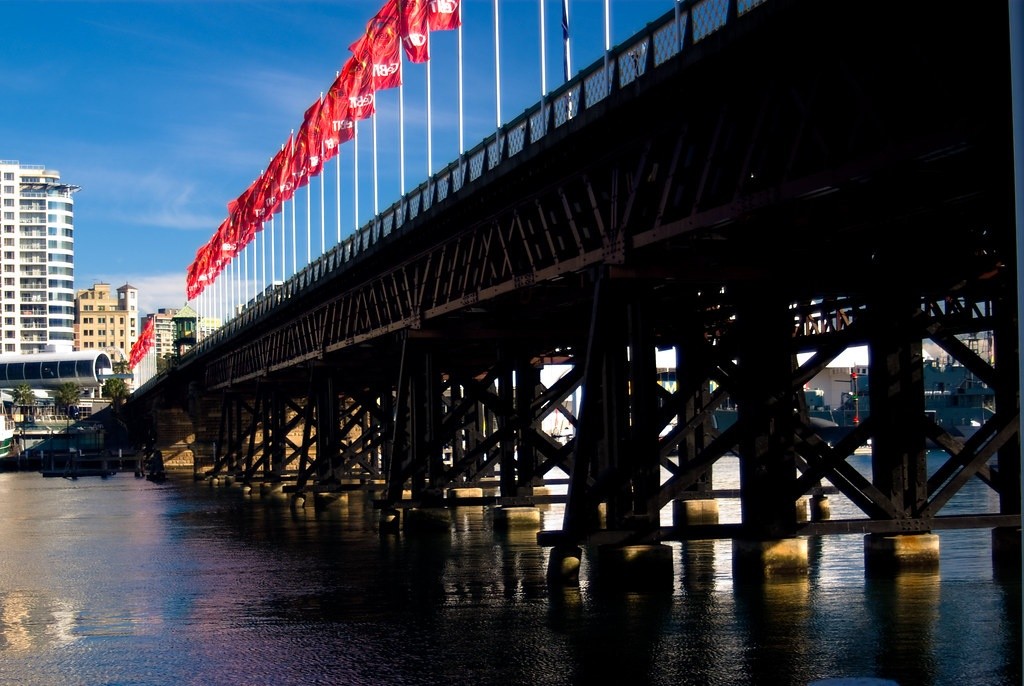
xmin=853 ymin=330 xmax=998 ymax=440
xmin=705 ymin=377 xmax=872 ymax=453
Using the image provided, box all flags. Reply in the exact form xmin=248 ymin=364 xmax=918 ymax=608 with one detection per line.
xmin=129 ymin=317 xmax=154 ymax=370
xmin=185 ymin=0 xmax=463 ymax=302
